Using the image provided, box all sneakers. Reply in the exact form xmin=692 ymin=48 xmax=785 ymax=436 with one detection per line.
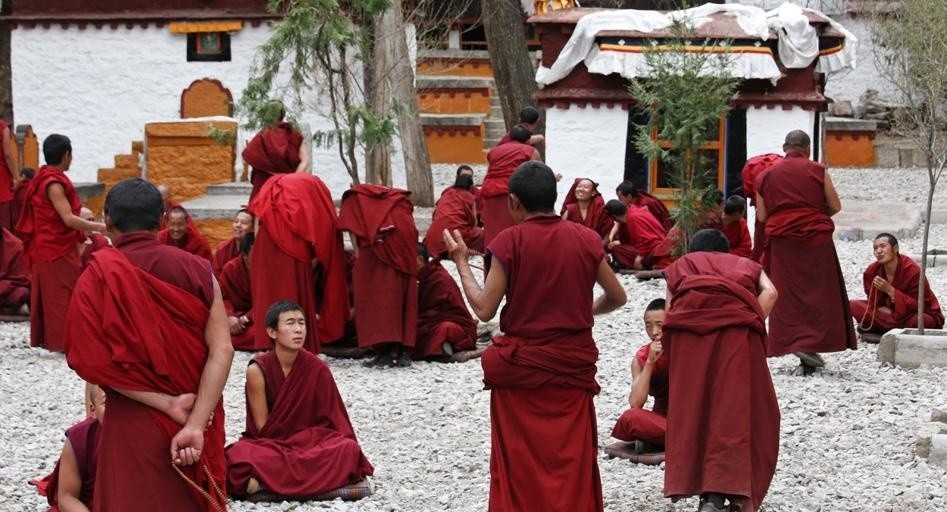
xmin=247 ymin=476 xmax=266 ymax=495
xmin=361 ymin=350 xmax=398 ymax=368
xmin=442 ymin=341 xmax=454 ymax=356
xmin=396 ymin=350 xmax=412 ymax=367
xmin=612 ymin=259 xmax=621 ymax=272
xmin=17 ymin=303 xmax=29 ymax=316
xmin=635 ymin=438 xmax=652 ymax=454
xmin=700 ymin=498 xmax=732 ymax=511
xmin=794 ymin=352 xmax=824 ymax=367
xmin=857 ymin=323 xmax=877 ymax=334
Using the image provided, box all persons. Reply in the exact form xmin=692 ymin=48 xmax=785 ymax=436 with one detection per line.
xmin=754 ymin=129 xmax=858 ymax=376
xmin=559 ymin=177 xmax=614 ymax=254
xmin=213 ymin=209 xmax=257 ymax=277
xmin=0 ymin=224 xmax=33 ymax=322
xmin=14 ymin=134 xmax=107 ymax=353
xmin=740 ymin=153 xmax=784 ymax=263
xmin=481 ymin=124 xmax=563 ymax=284
xmin=495 ymin=105 xmax=545 ymax=152
xmin=340 ymin=182 xmax=419 ymax=368
xmin=662 ymin=228 xmax=781 ymax=512
xmin=224 ymin=298 xmax=375 ymax=499
xmin=432 ymin=165 xmax=484 ymax=229
xmin=217 ymin=232 xmax=255 ymax=350
xmin=11 ymin=168 xmax=36 ymax=232
xmin=408 ymin=242 xmax=478 ymax=363
xmin=61 ymin=178 xmax=235 ymax=512
xmin=667 ymin=188 xmax=725 ymax=240
xmin=241 ymin=99 xmax=305 ymax=205
xmin=156 ymin=205 xmax=214 ymax=266
xmin=309 ymin=228 xmax=355 ymax=350
xmin=157 ymin=183 xmax=181 ymax=231
xmin=230 ymin=173 xmax=351 ymax=349
xmin=0 ymin=98 xmax=21 ymax=232
xmin=848 ymin=233 xmax=945 ymax=333
xmin=628 ymin=173 xmax=677 ymax=231
xmin=422 ymin=174 xmax=485 ymax=265
xmin=606 ymin=199 xmax=668 ymax=271
xmin=78 ymin=207 xmax=112 ymax=271
xmin=721 ymin=194 xmax=752 ymax=261
xmin=610 ymin=298 xmax=669 ymax=451
xmin=441 ymin=161 xmax=627 ymax=511
xmin=28 ymin=383 xmax=107 ymax=512
